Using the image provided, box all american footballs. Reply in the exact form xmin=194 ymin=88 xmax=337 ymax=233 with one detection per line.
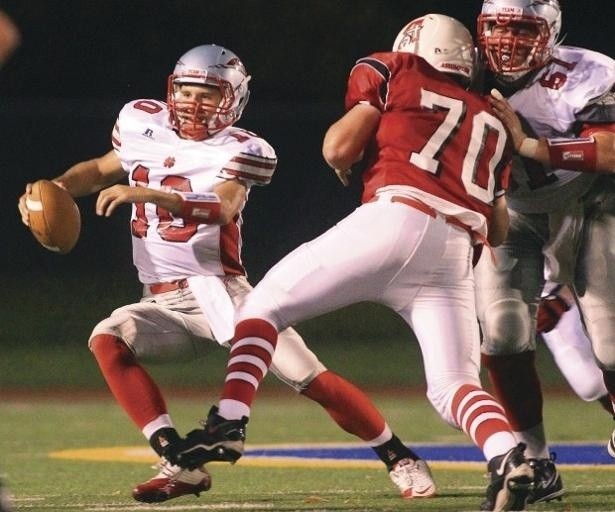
xmin=25 ymin=180 xmax=81 ymax=254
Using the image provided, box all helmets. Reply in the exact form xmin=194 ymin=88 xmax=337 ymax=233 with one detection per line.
xmin=165 ymin=45 xmax=254 ymax=142
xmin=391 ymin=14 xmax=477 ymax=91
xmin=474 ymin=1 xmax=565 ymax=79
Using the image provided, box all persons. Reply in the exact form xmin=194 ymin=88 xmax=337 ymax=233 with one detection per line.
xmin=17 ymin=41 xmax=439 ymax=504
xmin=473 ymin=255 xmax=615 ymax=417
xmin=470 ymin=0 xmax=615 ymax=509
xmin=163 ymin=12 xmax=537 ymax=512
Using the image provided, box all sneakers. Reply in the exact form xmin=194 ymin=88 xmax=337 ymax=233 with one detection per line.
xmin=164 ymin=405 xmax=250 ymax=471
xmin=527 ymin=452 xmax=565 ymax=505
xmin=131 ymin=459 xmax=214 ymax=504
xmin=480 ymin=444 xmax=535 ymax=512
xmin=388 ymin=456 xmax=436 ymax=501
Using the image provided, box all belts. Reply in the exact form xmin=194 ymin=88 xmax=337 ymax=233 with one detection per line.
xmin=150 ymin=280 xmax=189 ymax=293
xmin=366 ymin=193 xmax=501 ymax=267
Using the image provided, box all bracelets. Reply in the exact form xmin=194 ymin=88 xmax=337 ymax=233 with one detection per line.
xmin=518 ymin=136 xmax=539 ymax=159
